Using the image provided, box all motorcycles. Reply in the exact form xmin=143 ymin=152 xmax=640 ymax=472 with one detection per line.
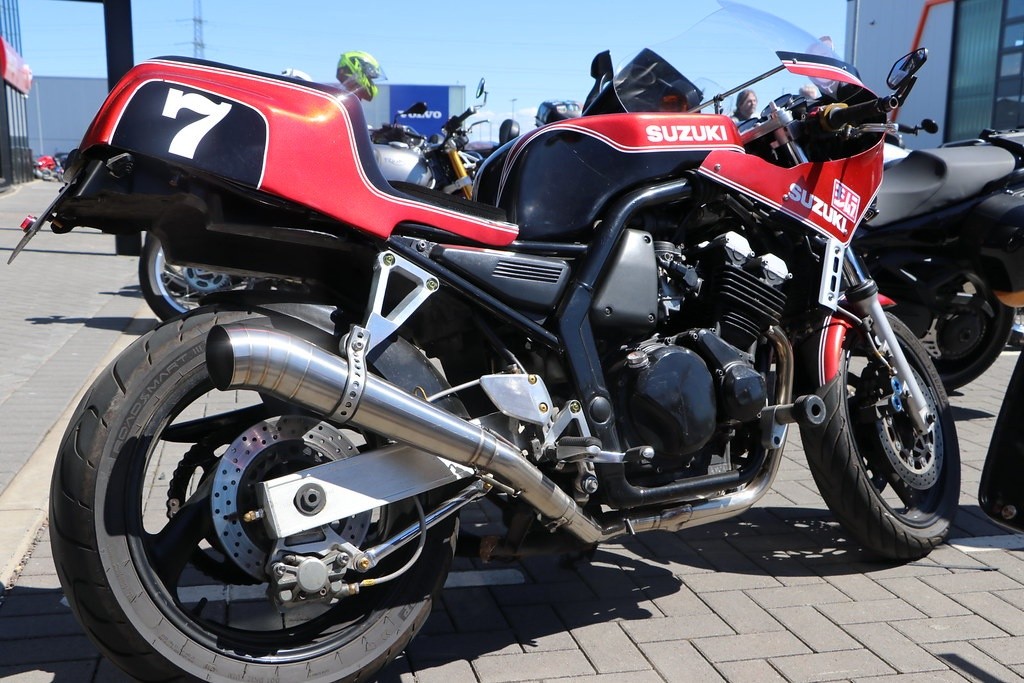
xmin=32 ymin=152 xmax=70 ymax=182
xmin=7 ymin=47 xmax=963 ymax=683
xmin=135 ymin=76 xmax=1024 ymax=393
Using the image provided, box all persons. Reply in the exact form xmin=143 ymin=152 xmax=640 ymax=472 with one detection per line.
xmin=730 ymin=90 xmax=757 ymax=124
xmin=799 ymin=85 xmax=816 ymax=99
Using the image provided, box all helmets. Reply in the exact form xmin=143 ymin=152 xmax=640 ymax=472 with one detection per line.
xmin=337 ymin=50 xmax=380 ymax=101
